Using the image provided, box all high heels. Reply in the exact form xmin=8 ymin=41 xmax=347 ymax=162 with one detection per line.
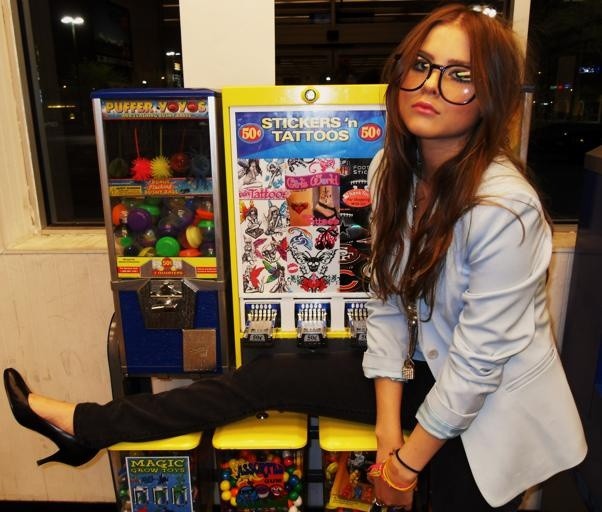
xmin=3 ymin=368 xmax=100 ymax=466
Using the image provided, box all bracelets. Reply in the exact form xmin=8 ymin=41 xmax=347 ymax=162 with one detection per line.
xmin=366 ymin=455 xmax=419 ymax=491
xmin=393 ymin=445 xmax=423 ymax=478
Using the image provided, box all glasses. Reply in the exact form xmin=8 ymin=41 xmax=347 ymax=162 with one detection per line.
xmin=390 ymin=54 xmax=482 ymax=104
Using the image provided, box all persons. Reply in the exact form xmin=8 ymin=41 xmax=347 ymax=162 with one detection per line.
xmin=5 ymin=5 xmax=589 ymax=510
xmin=239 ymin=157 xmax=290 ymax=294
